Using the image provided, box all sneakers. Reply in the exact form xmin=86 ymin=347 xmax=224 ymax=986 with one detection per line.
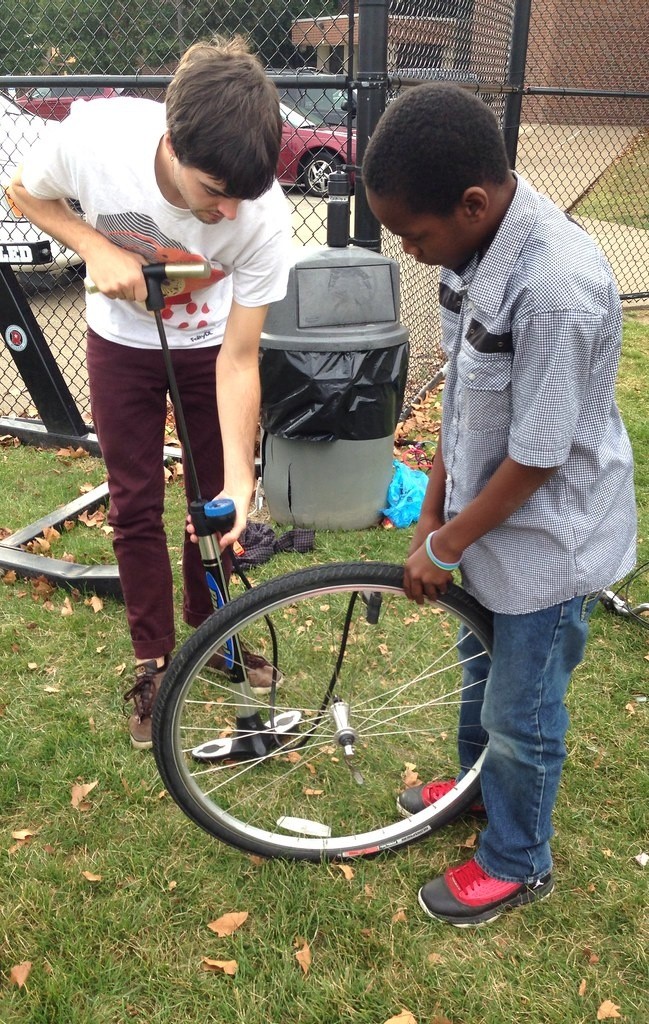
xmin=418 ymin=856 xmax=557 ymax=927
xmin=398 ymin=775 xmax=484 ymax=819
xmin=199 ymin=646 xmax=285 ymax=695
xmin=121 ymin=653 xmax=161 ymax=753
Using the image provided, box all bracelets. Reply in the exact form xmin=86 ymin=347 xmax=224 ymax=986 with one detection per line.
xmin=426 ymin=530 xmax=470 ymax=571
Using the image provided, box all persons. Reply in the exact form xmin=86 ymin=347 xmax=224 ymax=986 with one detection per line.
xmin=362 ymin=84 xmax=638 ymax=927
xmin=10 ymin=37 xmax=290 ymax=747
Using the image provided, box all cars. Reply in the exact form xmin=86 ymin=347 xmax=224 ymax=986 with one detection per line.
xmin=11 ymin=69 xmax=359 ymax=198
xmin=1 ymin=91 xmax=91 ymax=293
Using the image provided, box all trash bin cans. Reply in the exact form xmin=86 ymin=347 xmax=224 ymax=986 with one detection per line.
xmin=256 ymin=243 xmax=412 ymax=534
